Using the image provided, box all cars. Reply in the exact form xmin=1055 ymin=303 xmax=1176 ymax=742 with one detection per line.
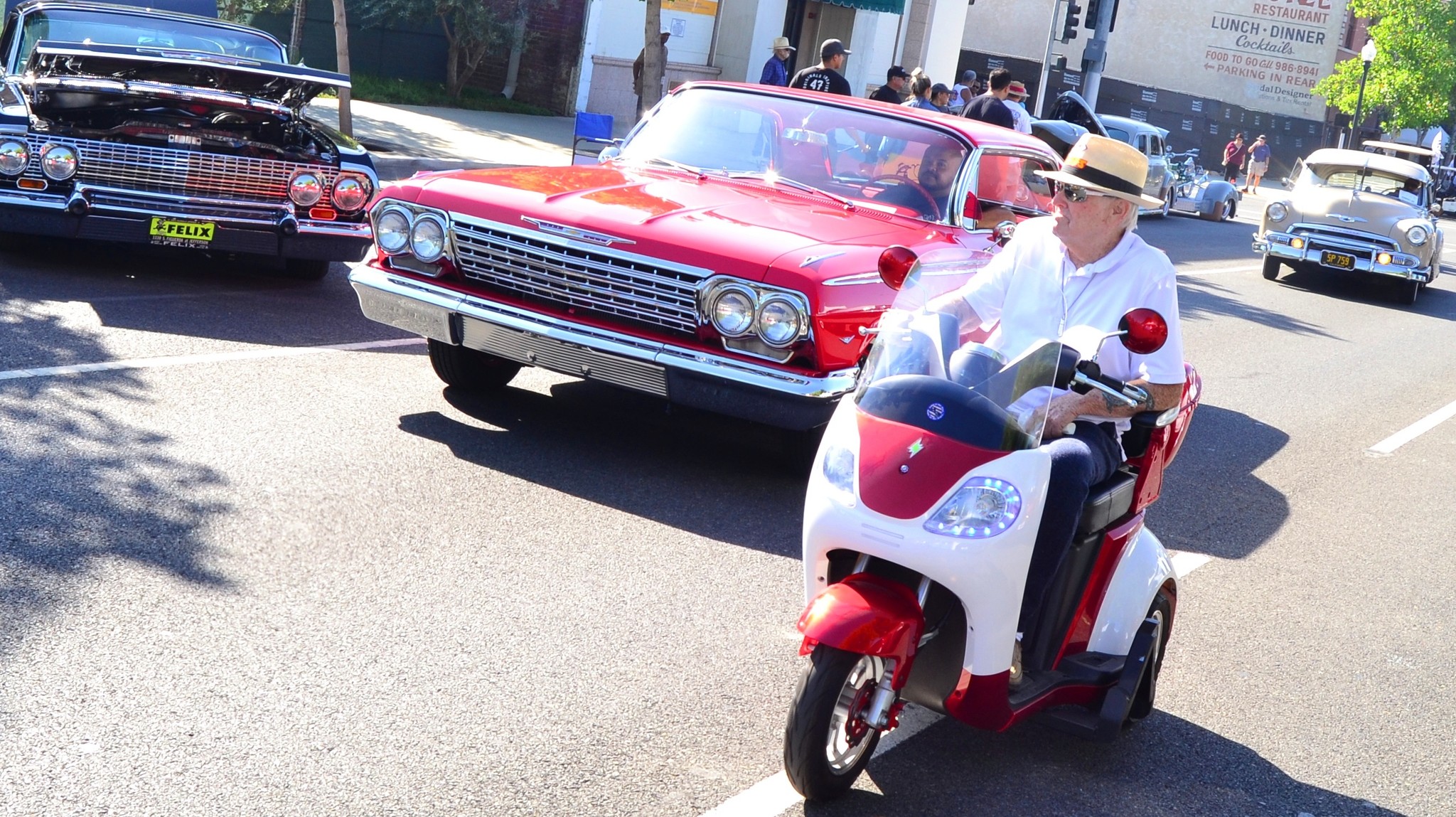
xmin=1250 ymin=148 xmax=1444 ymax=306
xmin=1030 ymin=90 xmax=1178 ymax=220
xmin=349 ymin=78 xmax=1063 ymax=476
xmin=0 ymin=1 xmax=380 ymax=295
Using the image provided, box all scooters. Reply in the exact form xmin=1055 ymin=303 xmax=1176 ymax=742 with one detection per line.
xmin=782 ymin=220 xmax=1203 ymax=800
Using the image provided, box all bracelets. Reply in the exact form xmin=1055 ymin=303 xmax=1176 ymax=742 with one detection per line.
xmin=1265 ymin=165 xmax=1268 ymax=168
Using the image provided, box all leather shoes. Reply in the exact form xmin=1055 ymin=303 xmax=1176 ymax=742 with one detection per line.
xmin=1008 ymin=639 xmax=1023 ymax=693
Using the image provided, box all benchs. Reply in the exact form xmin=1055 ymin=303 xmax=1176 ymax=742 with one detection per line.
xmin=816 ymin=180 xmax=1030 ymax=229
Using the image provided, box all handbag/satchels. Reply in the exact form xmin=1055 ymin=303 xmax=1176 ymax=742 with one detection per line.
xmin=1222 ymin=160 xmax=1227 ymax=166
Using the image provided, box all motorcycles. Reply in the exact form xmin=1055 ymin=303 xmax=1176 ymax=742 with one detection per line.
xmin=1165 ymin=145 xmax=1243 ymax=222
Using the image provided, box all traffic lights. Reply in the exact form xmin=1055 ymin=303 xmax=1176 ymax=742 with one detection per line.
xmin=1061 ymin=0 xmax=1082 ymax=45
xmin=1051 ymin=56 xmax=1067 ymax=70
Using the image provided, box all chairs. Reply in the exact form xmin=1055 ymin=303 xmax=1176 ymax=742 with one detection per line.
xmin=1074 ymin=401 xmax=1181 ymax=537
xmin=571 ymin=111 xmax=625 ymax=165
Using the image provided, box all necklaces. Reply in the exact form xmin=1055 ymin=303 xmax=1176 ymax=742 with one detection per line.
xmin=1059 ymin=260 xmax=1098 ymax=337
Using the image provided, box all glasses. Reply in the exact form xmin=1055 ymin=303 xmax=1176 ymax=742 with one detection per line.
xmin=1054 ymin=179 xmax=1123 ymax=204
xmin=783 ymin=48 xmax=789 ymax=51
xmin=902 ymin=77 xmax=905 ymax=80
xmin=973 ymin=85 xmax=980 ymax=90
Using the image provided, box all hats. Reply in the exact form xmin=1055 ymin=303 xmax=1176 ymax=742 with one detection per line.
xmin=1033 ymin=132 xmax=1165 ymax=210
xmin=1009 ymin=81 xmax=1031 ymax=98
xmin=932 ymin=83 xmax=956 ymax=94
xmin=1256 ymin=135 xmax=1267 ymax=141
xmin=820 ymin=38 xmax=852 ymax=56
xmin=661 ymin=25 xmax=671 ymax=35
xmin=768 ymin=37 xmax=796 ymax=51
xmin=888 ymin=65 xmax=910 ymax=77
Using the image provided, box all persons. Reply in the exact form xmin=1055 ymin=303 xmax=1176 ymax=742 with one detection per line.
xmin=869 ymin=65 xmax=911 ymax=105
xmin=899 ymin=66 xmax=940 ymax=112
xmin=875 ymin=132 xmax=1188 ymax=688
xmin=947 ymin=70 xmax=978 ymax=116
xmin=1240 ymin=134 xmax=1270 ymax=195
xmin=1384 ymin=177 xmax=1422 ymax=206
xmin=785 ymin=38 xmax=869 ymax=153
xmin=1222 ymin=133 xmax=1246 ymax=185
xmin=930 ymin=83 xmax=956 ymax=114
xmin=633 ymin=25 xmax=672 ymax=125
xmin=871 ymin=144 xmax=964 ymax=222
xmin=1000 ymin=80 xmax=1033 ymax=209
xmin=957 ymin=68 xmax=1015 ymax=199
xmin=968 ymin=77 xmax=981 ymax=98
xmin=759 ymin=37 xmax=796 ymax=87
xmin=1018 ymin=88 xmax=1028 ymax=111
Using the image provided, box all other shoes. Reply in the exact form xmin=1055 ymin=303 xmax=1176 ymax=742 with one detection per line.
xmin=1242 ymin=188 xmax=1248 ymax=193
xmin=1253 ymin=189 xmax=1256 ymax=194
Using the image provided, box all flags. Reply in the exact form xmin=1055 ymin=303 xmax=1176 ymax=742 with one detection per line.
xmin=1431 ymin=131 xmax=1445 ymax=163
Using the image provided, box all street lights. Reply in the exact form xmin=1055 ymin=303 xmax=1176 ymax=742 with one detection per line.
xmin=1346 ymin=39 xmax=1378 ymax=150
